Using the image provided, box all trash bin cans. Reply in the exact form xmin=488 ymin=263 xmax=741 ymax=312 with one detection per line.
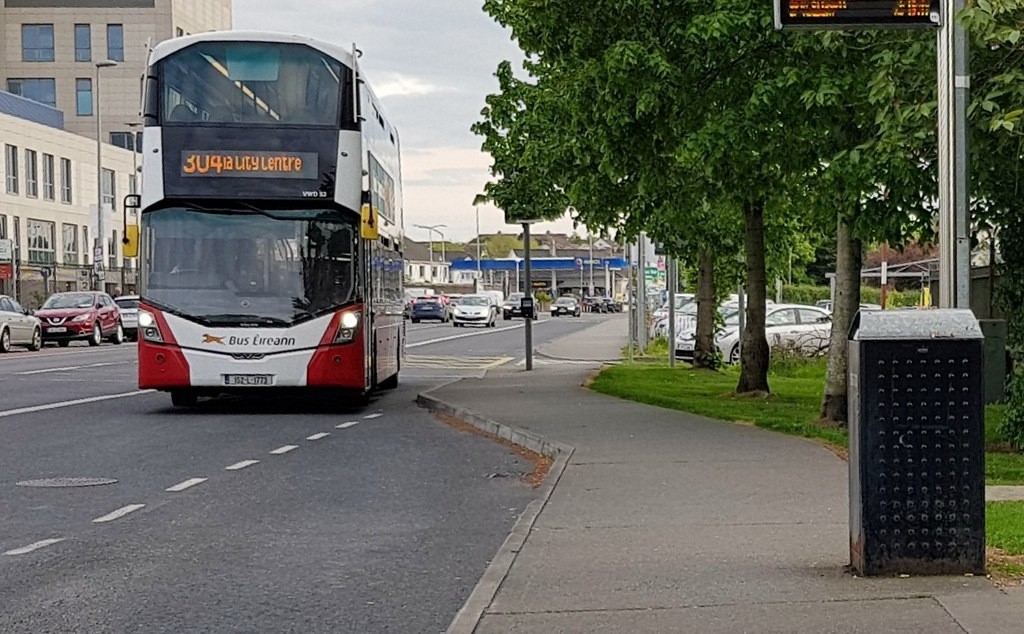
xmin=845 ymin=309 xmax=987 ymax=575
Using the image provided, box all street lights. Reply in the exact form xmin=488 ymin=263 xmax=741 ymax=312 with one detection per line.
xmin=94 ymin=60 xmax=118 ymax=294
xmin=429 ymin=224 xmax=448 ymax=285
xmin=120 ymin=29 xmax=405 ymax=407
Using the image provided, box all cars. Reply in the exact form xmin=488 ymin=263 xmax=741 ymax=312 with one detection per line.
xmin=674 ymin=303 xmax=833 ymax=367
xmin=443 ymin=294 xmax=465 ymax=319
xmin=502 ymin=292 xmax=539 ymax=320
xmin=452 ymin=293 xmax=499 ymax=328
xmin=549 ymin=297 xmax=581 ymax=318
xmin=410 ymin=295 xmax=450 ymax=323
xmin=32 ymin=291 xmax=124 ymax=347
xmin=651 ymin=292 xmax=787 ymax=344
xmin=0 ymin=295 xmax=43 ymax=353
xmin=112 ymin=295 xmax=141 ymax=343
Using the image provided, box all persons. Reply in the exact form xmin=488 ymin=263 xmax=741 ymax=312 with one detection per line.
xmin=129 ymin=289 xmax=136 ymax=295
xmin=112 ymin=287 xmax=122 ymax=300
xmin=171 ymin=236 xmax=239 ymax=293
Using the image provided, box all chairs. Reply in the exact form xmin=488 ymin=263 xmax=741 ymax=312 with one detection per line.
xmin=208 ymin=105 xmax=234 ymax=122
xmin=171 ymin=104 xmax=195 ymax=120
